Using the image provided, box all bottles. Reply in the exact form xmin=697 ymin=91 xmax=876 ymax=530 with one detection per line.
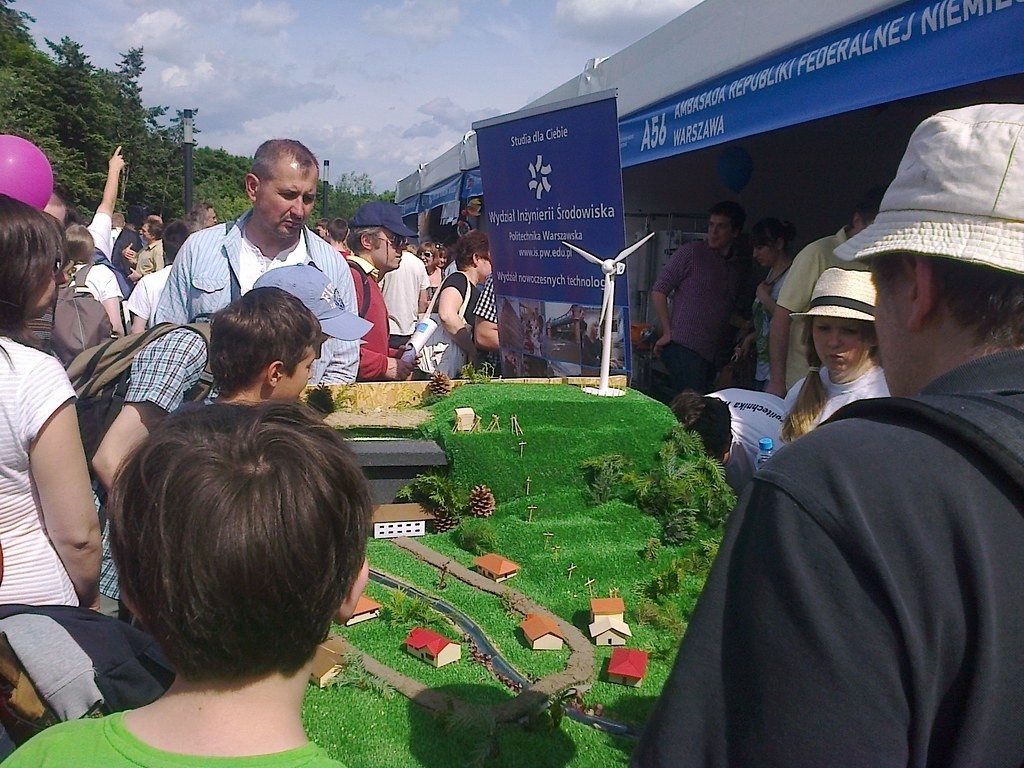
xmin=754 ymin=438 xmax=778 ymax=471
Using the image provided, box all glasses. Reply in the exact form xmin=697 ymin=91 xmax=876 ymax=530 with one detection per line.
xmin=420 ymin=253 xmax=433 ymax=258
xmin=377 ymin=237 xmax=406 ymax=250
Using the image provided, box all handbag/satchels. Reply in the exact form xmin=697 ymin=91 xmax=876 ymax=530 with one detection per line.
xmin=405 ymin=271 xmax=471 ymax=380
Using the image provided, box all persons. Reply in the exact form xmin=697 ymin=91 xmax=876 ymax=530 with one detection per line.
xmin=0 ymin=138 xmax=501 ymax=768
xmin=650 ymin=185 xmax=891 ymax=500
xmin=636 ymin=102 xmax=1024 ymax=768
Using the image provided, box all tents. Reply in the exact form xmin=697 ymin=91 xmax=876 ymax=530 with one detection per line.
xmin=393 ymin=0 xmax=1023 ymax=385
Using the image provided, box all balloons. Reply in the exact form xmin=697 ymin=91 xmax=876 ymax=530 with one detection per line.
xmin=0 ymin=134 xmax=52 ymax=211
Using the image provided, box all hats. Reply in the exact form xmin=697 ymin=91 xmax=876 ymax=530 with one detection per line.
xmin=254 ymin=264 xmax=374 ymax=341
xmin=833 ymin=104 xmax=1024 ymax=275
xmin=348 ymin=201 xmax=410 ymax=237
xmin=789 ymin=268 xmax=877 ymax=321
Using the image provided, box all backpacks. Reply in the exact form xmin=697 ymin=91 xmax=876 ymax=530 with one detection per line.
xmin=54 ymin=264 xmax=114 ymax=371
xmin=68 ymin=323 xmax=214 ymax=479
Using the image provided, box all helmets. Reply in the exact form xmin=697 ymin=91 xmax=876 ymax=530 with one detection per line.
xmin=0 ymin=134 xmax=54 ymax=211
